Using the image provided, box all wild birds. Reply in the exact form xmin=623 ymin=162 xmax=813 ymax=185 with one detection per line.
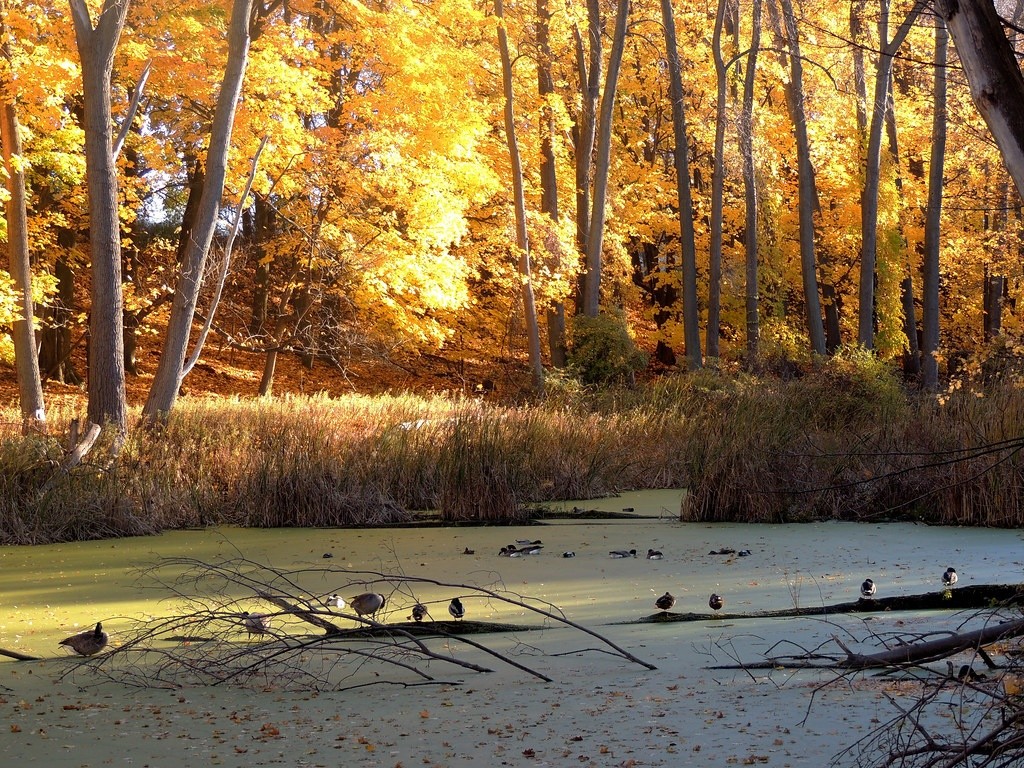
xmin=562 ymin=551 xmax=575 ymax=558
xmin=238 ymin=611 xmax=270 ymax=643
xmin=737 ymin=548 xmax=752 ymax=557
xmin=324 ymin=592 xmax=467 ymax=630
xmin=709 ymin=594 xmax=723 ymax=616
xmin=941 ymin=567 xmax=958 ymax=590
xmin=498 ymin=539 xmax=544 ymax=557
xmin=861 ymin=578 xmax=877 ymax=600
xmin=608 ymin=549 xmax=663 ymax=561
xmin=653 ymin=591 xmax=675 ymax=611
xmin=58 ymin=621 xmax=108 ymax=658
